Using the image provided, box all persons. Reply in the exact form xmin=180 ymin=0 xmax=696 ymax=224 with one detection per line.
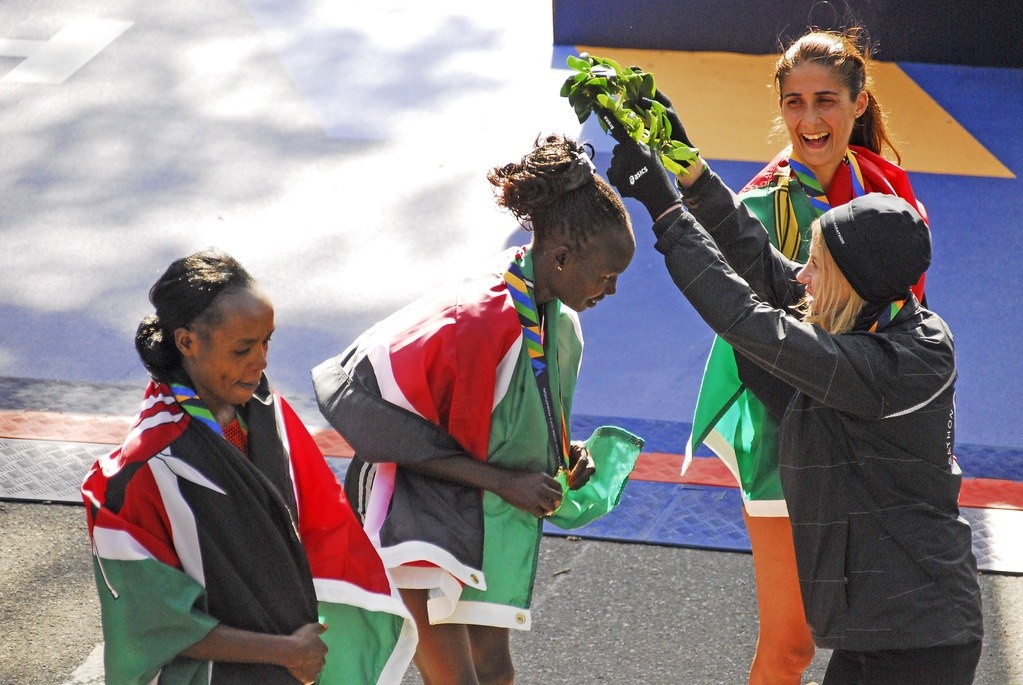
xmin=592 ymin=67 xmax=984 ymax=685
xmin=308 ymin=131 xmax=645 ymax=685
xmin=681 ymin=26 xmax=930 ymax=685
xmin=80 ymin=252 xmax=419 ymax=685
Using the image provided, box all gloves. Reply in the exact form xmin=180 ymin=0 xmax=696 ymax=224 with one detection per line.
xmin=629 ymin=66 xmax=700 ymax=168
xmin=599 ymin=109 xmax=682 ymax=222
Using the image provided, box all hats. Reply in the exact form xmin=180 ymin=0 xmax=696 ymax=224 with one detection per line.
xmin=820 ymin=192 xmax=932 ymax=303
